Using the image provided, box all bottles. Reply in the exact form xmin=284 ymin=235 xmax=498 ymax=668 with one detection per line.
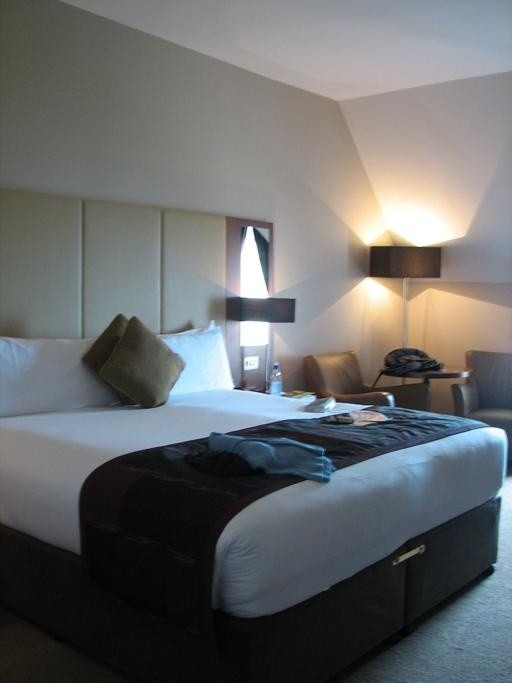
xmin=270 ymin=364 xmax=282 ymax=398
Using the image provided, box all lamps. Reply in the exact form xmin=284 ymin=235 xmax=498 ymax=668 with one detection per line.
xmin=369 ymin=245 xmax=440 ymax=368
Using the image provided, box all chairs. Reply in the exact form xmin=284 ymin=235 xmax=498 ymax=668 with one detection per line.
xmin=301 ymin=350 xmax=512 ymax=477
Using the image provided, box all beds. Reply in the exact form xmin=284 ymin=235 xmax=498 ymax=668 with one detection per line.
xmin=0 ymin=323 xmax=508 ymax=682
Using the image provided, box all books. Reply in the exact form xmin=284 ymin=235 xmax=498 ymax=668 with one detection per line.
xmin=304 ymin=396 xmax=335 ymax=412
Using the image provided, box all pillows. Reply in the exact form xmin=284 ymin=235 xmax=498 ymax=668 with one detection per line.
xmin=0 ymin=313 xmax=237 ymax=417
